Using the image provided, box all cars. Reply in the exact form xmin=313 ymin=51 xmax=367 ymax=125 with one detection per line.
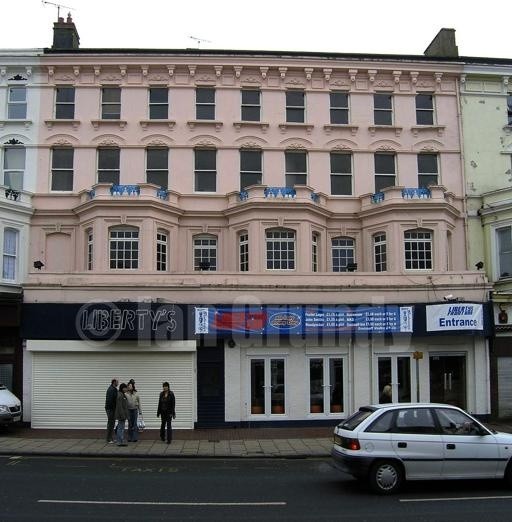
xmin=331 ymin=402 xmax=512 ymax=495
xmin=0 ymin=382 xmax=22 ymax=436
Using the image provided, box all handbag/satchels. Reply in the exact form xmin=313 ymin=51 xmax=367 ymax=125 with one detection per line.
xmin=139 ymin=421 xmax=145 ymax=428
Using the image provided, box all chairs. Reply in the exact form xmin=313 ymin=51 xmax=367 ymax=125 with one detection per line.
xmin=416 ymin=415 xmax=432 ymax=434
xmin=403 ymin=413 xmax=416 ymax=425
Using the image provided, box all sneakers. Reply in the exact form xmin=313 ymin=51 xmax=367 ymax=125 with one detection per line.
xmin=167 ymin=441 xmax=171 ymax=444
xmin=108 ymin=440 xmax=137 ymax=447
xmin=161 ymin=438 xmax=165 ymax=442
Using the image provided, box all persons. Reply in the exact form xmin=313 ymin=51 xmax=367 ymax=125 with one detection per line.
xmin=115 ymin=382 xmax=131 ymax=446
xmin=124 ymin=382 xmax=142 ymax=442
xmin=104 ymin=378 xmax=118 ymax=443
xmin=379 ymin=384 xmax=393 ymax=404
xmin=156 ymin=382 xmax=176 ymax=444
